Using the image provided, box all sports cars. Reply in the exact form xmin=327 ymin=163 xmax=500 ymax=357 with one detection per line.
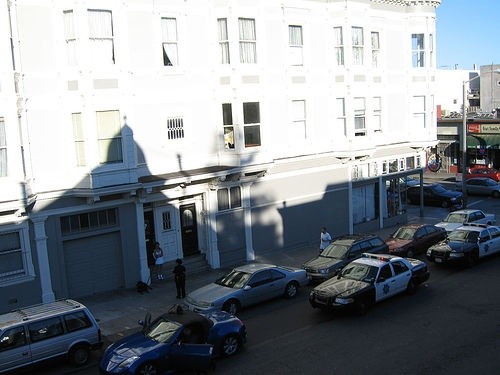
xmin=99 ymin=308 xmax=248 ymax=375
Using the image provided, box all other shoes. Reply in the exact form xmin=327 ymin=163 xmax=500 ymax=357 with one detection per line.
xmin=158 ymin=274 xmax=163 ymax=279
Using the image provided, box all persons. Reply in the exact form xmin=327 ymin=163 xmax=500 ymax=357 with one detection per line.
xmin=319 ymin=228 xmax=332 ymax=253
xmin=153 ymin=242 xmax=165 ymax=280
xmin=173 ymin=260 xmax=186 ymax=299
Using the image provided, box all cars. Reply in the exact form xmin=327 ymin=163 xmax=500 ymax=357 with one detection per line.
xmin=308 ymin=253 xmax=430 ymax=315
xmin=183 ymin=263 xmax=308 ymax=317
xmin=384 ymin=223 xmax=447 ymax=258
xmin=465 ymin=165 xmax=500 ymax=183
xmin=386 ymin=176 xmax=462 ymax=208
xmin=434 ymin=210 xmax=496 ymax=236
xmin=425 ymin=223 xmax=500 ymax=267
xmin=455 ymin=177 xmax=500 ymax=198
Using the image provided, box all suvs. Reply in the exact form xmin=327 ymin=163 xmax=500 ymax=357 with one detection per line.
xmin=0 ymin=298 xmax=105 ymax=375
xmin=302 ymin=233 xmax=389 ymax=286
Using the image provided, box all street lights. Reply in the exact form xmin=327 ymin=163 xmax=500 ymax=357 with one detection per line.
xmin=461 ymin=69 xmax=500 ymax=210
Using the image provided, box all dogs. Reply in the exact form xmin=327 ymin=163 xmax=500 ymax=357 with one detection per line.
xmin=135 ymin=280 xmax=153 ymax=296
